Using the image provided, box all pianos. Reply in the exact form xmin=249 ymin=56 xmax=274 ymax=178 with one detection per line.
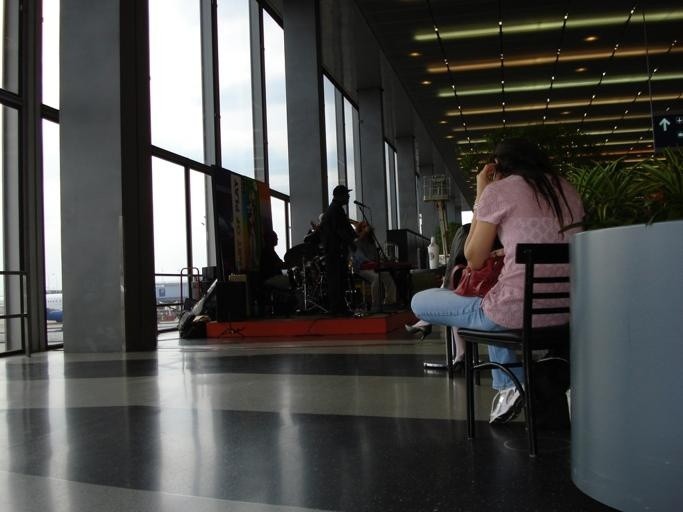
xmin=360 ymin=260 xmax=417 ymax=272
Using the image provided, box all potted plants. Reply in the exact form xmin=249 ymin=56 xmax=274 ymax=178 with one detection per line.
xmin=569 ymin=147 xmax=683 ymax=511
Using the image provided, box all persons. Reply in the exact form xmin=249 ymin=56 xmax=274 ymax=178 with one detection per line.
xmin=409 ymin=138 xmax=587 ymax=426
xmin=319 ymin=184 xmax=358 ymax=316
xmin=349 ymin=219 xmax=406 ymax=314
xmin=261 ymin=230 xmax=296 ymax=318
xmin=402 ymin=222 xmax=504 ymax=373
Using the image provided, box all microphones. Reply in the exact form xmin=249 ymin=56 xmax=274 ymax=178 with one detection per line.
xmin=354 ymin=200 xmax=368 ymax=208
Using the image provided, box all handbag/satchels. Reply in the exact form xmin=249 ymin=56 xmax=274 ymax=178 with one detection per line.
xmin=452 ymin=250 xmax=505 ymax=298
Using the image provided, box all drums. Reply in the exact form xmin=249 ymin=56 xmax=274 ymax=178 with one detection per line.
xmin=312 ymin=254 xmax=329 ymax=278
xmin=287 ymin=260 xmax=321 ymax=295
xmin=308 ymin=272 xmax=329 ymax=313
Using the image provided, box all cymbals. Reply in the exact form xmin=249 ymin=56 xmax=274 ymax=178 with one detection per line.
xmin=284 ymin=243 xmax=317 ymax=266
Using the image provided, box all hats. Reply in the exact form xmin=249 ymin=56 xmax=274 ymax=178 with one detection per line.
xmin=332 ymin=185 xmax=352 ymax=195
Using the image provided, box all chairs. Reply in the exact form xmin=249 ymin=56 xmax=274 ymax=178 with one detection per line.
xmin=457 ymin=243 xmax=569 ymax=461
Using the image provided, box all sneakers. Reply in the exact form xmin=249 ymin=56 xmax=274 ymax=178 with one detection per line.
xmin=487 ymin=379 xmax=525 ymax=426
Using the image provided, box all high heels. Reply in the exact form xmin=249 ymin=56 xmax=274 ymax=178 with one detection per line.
xmin=403 ymin=321 xmax=431 ymax=340
xmin=446 ymin=353 xmax=465 ymax=374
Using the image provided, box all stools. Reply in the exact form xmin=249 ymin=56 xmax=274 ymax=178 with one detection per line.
xmin=353 ymin=277 xmax=371 ymax=309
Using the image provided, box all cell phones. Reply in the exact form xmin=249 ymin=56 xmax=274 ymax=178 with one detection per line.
xmin=488 ymin=164 xmax=503 ymax=180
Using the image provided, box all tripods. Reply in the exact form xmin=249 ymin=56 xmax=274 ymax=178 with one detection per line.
xmin=294 ymin=270 xmax=328 ymax=313
xmin=216 ymin=238 xmax=245 ymax=339
